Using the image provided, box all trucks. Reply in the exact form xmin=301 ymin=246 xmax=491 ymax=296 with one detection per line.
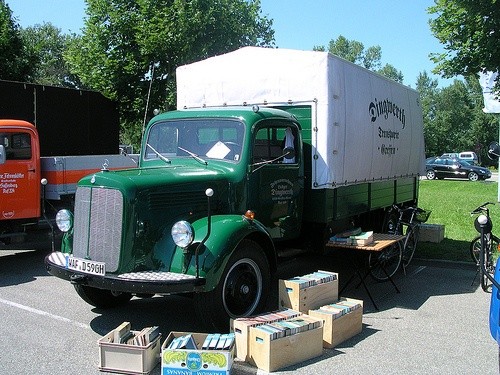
xmin=0 ymin=118 xmax=177 ymax=257
xmin=43 ymin=46 xmax=426 ymax=334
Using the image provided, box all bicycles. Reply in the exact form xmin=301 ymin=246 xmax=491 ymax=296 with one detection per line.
xmin=470 ymin=202 xmax=500 ymax=292
xmin=367 ymin=202 xmax=432 ymax=283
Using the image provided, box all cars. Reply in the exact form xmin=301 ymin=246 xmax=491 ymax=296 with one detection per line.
xmin=424 ymin=156 xmax=492 ymax=182
xmin=441 ymin=152 xmax=480 ymax=166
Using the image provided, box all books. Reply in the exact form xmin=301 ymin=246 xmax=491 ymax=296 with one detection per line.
xmin=315 ymin=301 xmax=360 ymax=319
xmin=285 ymin=271 xmax=336 ymax=291
xmin=113 ymin=322 xmax=159 ymax=346
xmin=234 ymin=307 xmax=321 ymax=342
xmin=328 ymin=228 xmax=374 ymax=247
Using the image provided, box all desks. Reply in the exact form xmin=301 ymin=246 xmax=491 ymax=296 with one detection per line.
xmin=325 ymin=233 xmax=407 ymax=312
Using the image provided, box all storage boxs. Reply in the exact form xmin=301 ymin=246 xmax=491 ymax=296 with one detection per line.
xmin=97 ymin=270 xmax=364 ymax=375
xmin=418 ymin=224 xmax=445 ymax=244
